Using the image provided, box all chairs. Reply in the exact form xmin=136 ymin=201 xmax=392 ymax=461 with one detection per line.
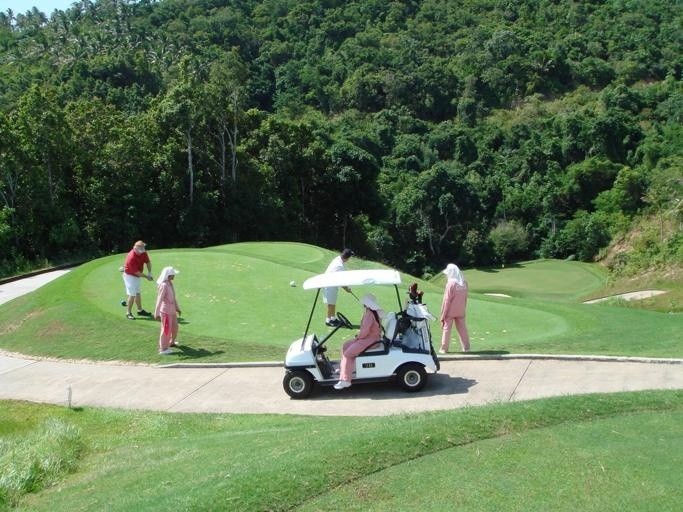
xmin=384 ymin=312 xmax=397 ymax=334
xmin=359 ymin=318 xmax=399 ymax=356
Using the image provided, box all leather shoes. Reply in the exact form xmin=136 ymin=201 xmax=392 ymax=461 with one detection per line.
xmin=325 ymin=319 xmax=341 ymax=327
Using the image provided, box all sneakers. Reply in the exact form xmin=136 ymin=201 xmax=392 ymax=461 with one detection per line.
xmin=127 ymin=313 xmax=135 ymax=319
xmin=170 ymin=342 xmax=178 ymax=345
xmin=334 ymin=380 xmax=351 ymax=389
xmin=137 ymin=310 xmax=151 ymax=316
xmin=160 ymin=349 xmax=173 ymax=354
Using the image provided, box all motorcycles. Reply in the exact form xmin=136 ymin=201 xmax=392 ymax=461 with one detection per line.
xmin=282 ymin=269 xmax=439 ymax=399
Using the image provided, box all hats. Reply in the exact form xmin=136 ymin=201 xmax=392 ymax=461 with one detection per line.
xmin=359 ymin=293 xmax=385 ymax=318
xmin=163 ymin=266 xmax=178 ymax=276
xmin=344 ymin=248 xmax=354 ymax=255
xmin=443 ymin=263 xmax=465 ymax=286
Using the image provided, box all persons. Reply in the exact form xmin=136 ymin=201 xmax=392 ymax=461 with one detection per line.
xmin=153 ymin=265 xmax=182 ymax=354
xmin=437 ymin=263 xmax=471 ymax=355
xmin=122 ymin=240 xmax=152 ymax=320
xmin=321 ymin=248 xmax=355 ymax=328
xmin=332 ymin=291 xmax=384 ymax=390
